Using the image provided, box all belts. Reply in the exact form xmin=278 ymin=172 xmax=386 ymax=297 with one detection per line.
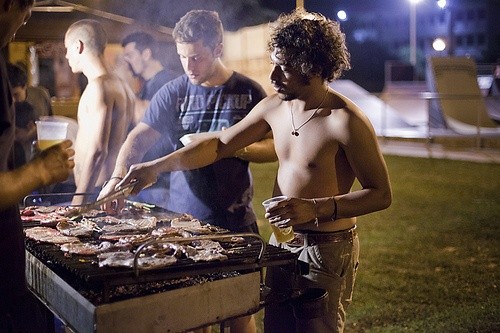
xmin=286 ymin=224 xmax=357 ymax=247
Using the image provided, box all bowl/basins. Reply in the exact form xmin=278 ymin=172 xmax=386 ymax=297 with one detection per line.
xmin=179 ymin=130 xmax=219 ymax=147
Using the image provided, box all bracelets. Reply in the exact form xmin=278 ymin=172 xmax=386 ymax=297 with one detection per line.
xmin=101 ymin=177 xmax=123 ymax=190
xmin=312 ymin=198 xmax=319 ymax=227
xmin=238 ymin=147 xmax=247 ymax=157
xmin=329 ymin=198 xmax=337 ymax=222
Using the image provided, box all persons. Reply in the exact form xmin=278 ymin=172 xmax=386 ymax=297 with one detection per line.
xmin=63 ymin=18 xmax=137 ymax=207
xmin=96 ymin=8 xmax=277 ymax=332
xmin=111 ymin=30 xmax=181 ymax=206
xmin=116 ymin=6 xmax=393 ymax=333
xmin=0 ymin=0 xmax=76 ymax=333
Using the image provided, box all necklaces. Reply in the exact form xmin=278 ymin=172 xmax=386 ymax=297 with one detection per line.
xmin=290 ymin=87 xmax=329 ymax=136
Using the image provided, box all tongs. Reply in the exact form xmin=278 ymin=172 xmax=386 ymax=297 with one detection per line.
xmin=64 ymin=178 xmax=157 ymax=219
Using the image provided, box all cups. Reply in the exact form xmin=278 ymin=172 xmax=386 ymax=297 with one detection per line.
xmin=262 ymin=195 xmax=295 ymax=243
xmin=34 ymin=121 xmax=68 ymax=150
